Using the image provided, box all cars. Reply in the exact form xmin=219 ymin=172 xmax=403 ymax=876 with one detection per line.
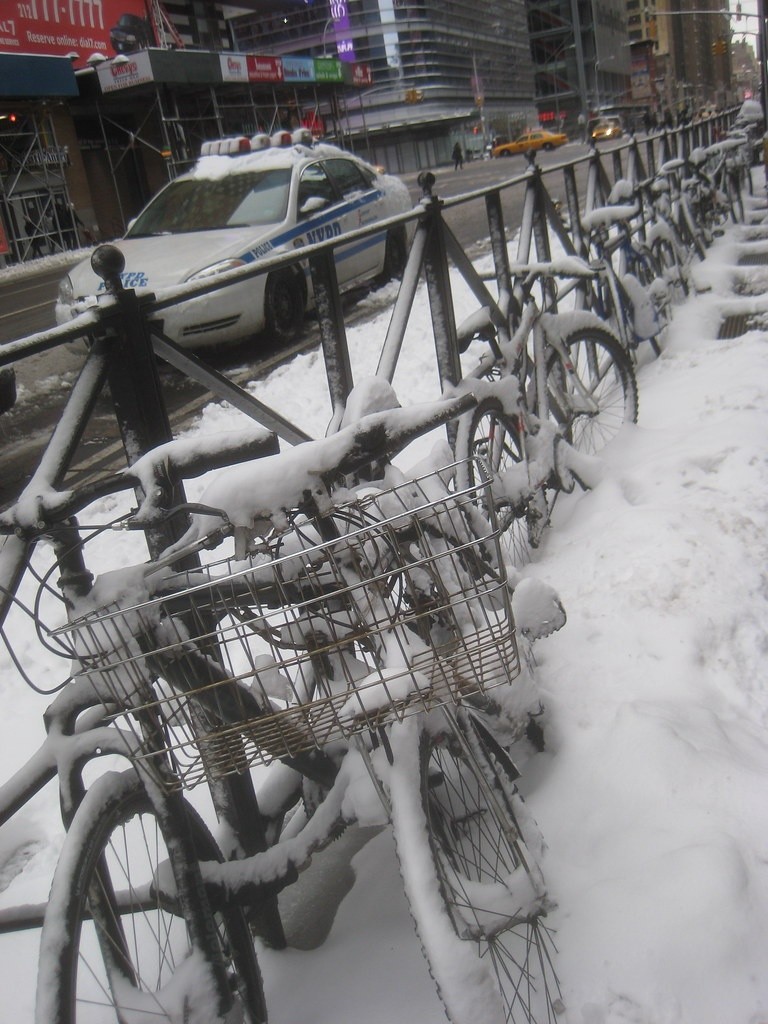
xmin=592 ymin=121 xmax=622 ymax=141
xmin=54 ymin=127 xmax=416 ymax=351
xmin=492 ymin=130 xmax=569 ymax=157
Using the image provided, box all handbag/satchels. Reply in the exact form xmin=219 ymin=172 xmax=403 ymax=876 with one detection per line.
xmin=452 ymin=151 xmax=455 ymax=159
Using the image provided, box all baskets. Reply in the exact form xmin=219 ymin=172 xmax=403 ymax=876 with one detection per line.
xmin=48 ymin=456 xmax=520 ymax=796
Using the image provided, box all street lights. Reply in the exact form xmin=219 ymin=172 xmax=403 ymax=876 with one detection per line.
xmin=594 ymin=54 xmax=616 ymax=115
xmin=471 ymin=19 xmax=500 ymax=155
xmin=553 ymin=43 xmax=578 ymax=132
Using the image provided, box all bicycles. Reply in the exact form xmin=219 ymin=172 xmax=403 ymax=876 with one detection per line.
xmin=581 ymin=114 xmax=768 ymax=367
xmin=450 ymin=255 xmax=641 ymax=586
xmin=0 ymin=388 xmax=571 ymax=1024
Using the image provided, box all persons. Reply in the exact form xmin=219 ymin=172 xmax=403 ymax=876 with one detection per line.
xmin=51 ymin=202 xmax=85 ymax=251
xmin=642 ymin=106 xmax=653 ymax=136
xmin=660 ymin=107 xmax=673 ymax=131
xmin=23 ymin=214 xmax=44 ymax=259
xmin=452 ymin=143 xmax=463 ymax=171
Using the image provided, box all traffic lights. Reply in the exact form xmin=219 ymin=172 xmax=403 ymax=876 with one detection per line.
xmin=412 ymin=89 xmax=423 ymax=104
xmin=719 ymin=40 xmax=727 ymax=55
xmin=711 ymin=41 xmax=719 ymax=55
xmin=405 ymin=87 xmax=412 ymax=104
